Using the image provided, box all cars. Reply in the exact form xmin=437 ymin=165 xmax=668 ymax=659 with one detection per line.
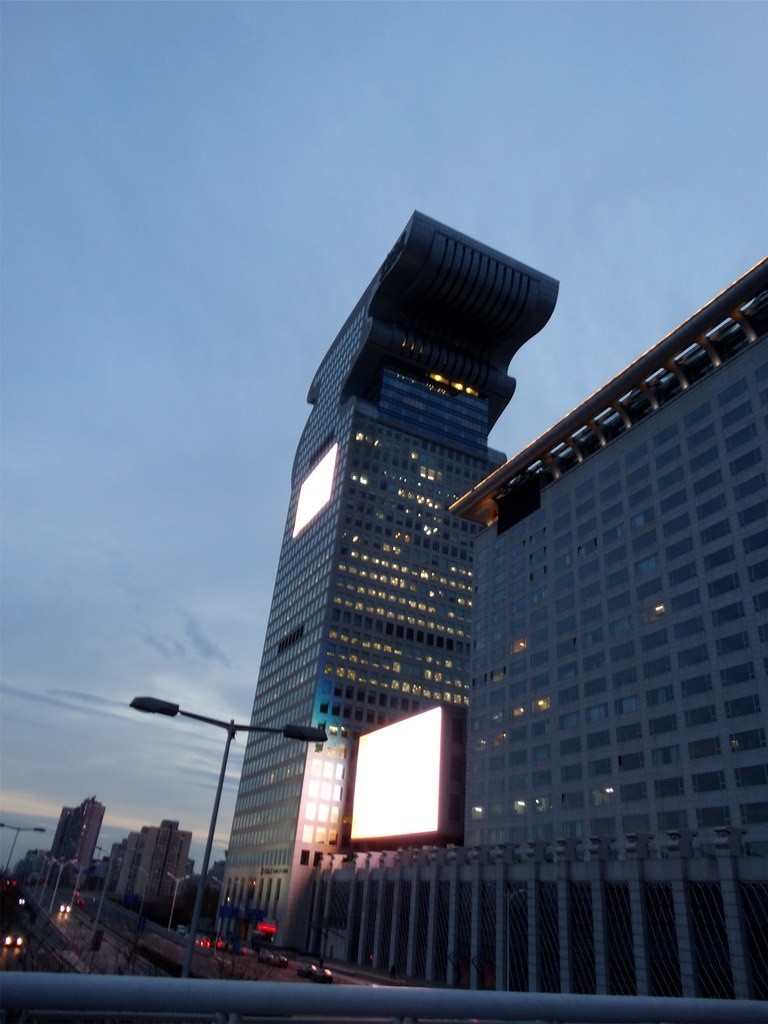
xmin=259 ymin=948 xmax=289 ymax=967
xmin=297 ymin=960 xmax=334 ymax=984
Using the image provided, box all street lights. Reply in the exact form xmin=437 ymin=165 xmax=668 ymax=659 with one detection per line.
xmin=0 ymin=823 xmax=46 ymax=870
xmin=128 ymin=695 xmax=328 ymax=977
xmin=167 ymin=871 xmax=191 ymax=929
xmin=43 ymin=855 xmax=102 ymax=934
